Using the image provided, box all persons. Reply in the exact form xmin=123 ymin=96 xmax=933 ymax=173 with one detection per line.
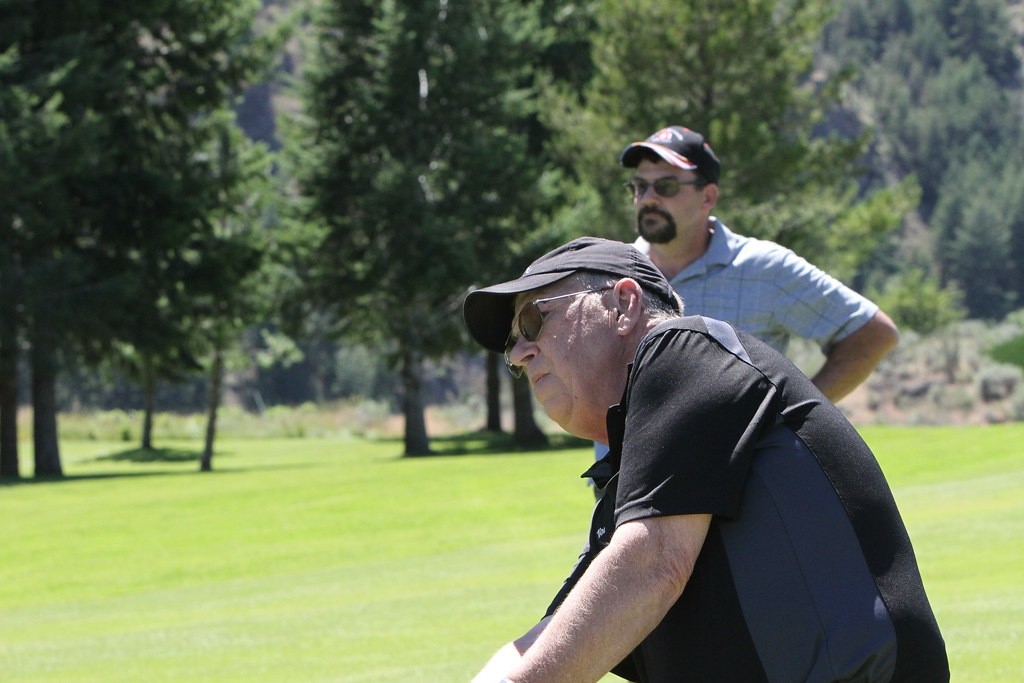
xmin=586 ymin=123 xmax=902 ymax=503
xmin=466 ymin=236 xmax=950 ymax=683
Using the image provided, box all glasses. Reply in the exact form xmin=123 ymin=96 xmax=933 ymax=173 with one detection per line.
xmin=503 ymin=285 xmax=615 ymax=380
xmin=623 ymin=175 xmax=709 ymax=197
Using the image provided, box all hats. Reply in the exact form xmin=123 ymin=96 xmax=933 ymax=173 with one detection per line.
xmin=461 ymin=236 xmax=685 ymax=354
xmin=621 ymin=124 xmax=721 ymax=186
